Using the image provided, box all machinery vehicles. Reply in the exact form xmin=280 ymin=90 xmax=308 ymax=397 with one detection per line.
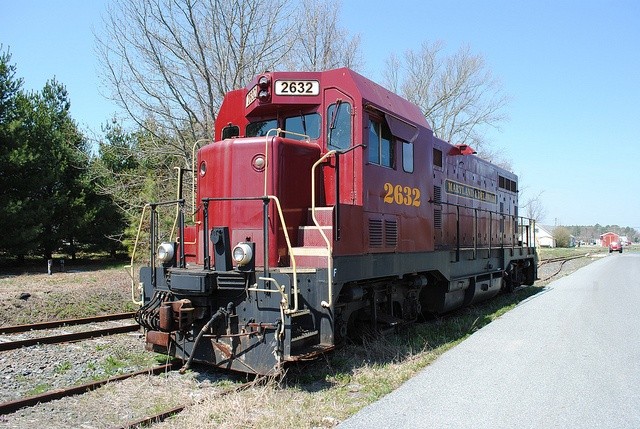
xmin=608 ymin=236 xmax=622 ymax=253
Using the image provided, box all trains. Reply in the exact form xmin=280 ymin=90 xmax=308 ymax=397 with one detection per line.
xmin=124 ymin=59 xmax=546 ymax=385
